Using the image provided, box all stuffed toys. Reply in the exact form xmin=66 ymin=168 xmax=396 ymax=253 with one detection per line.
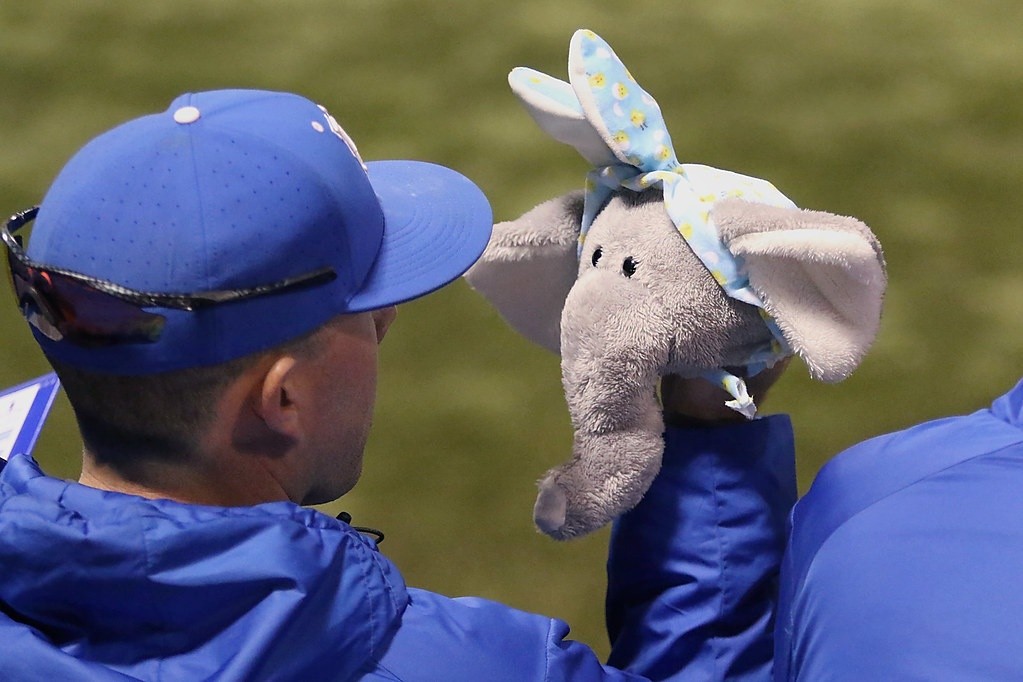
xmin=466 ymin=29 xmax=888 ymax=539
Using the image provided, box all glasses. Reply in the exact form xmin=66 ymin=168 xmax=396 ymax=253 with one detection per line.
xmin=0 ymin=203 xmax=338 ymax=353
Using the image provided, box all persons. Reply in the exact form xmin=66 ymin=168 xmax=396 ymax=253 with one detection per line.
xmin=775 ymin=378 xmax=1023 ymax=682
xmin=0 ymin=88 xmax=797 ymax=682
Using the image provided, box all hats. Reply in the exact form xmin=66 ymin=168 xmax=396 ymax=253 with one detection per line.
xmin=27 ymin=89 xmax=493 ymax=377
